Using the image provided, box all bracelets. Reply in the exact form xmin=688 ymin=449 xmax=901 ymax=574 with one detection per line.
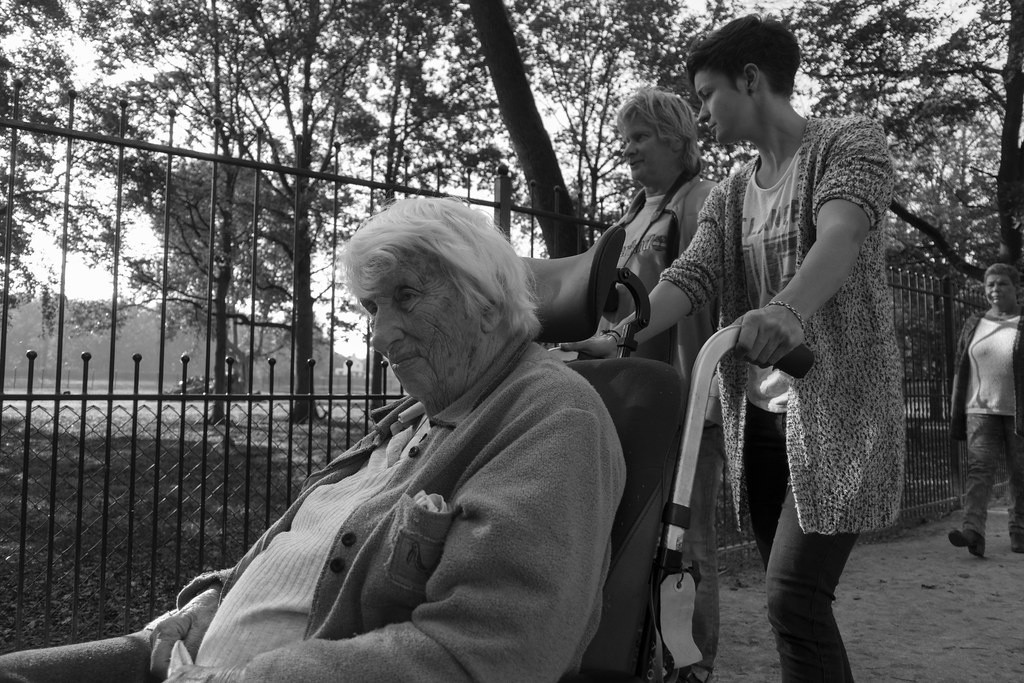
xmin=600 ymin=328 xmax=622 ymax=344
xmin=764 ymin=300 xmax=807 ymax=331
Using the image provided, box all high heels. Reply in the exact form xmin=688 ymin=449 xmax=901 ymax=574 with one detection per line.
xmin=1009 ymin=532 xmax=1024 ymax=552
xmin=948 ymin=529 xmax=985 ymax=557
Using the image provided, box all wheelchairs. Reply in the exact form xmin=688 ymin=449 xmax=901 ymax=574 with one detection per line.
xmin=511 ymin=223 xmax=815 ymax=683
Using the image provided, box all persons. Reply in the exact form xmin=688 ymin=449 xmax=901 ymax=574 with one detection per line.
xmin=946 ymin=262 xmax=1024 ymax=556
xmin=148 ymin=192 xmax=626 ymax=683
xmin=548 ymin=12 xmax=906 ymax=683
xmin=600 ymin=87 xmax=727 ymax=683
